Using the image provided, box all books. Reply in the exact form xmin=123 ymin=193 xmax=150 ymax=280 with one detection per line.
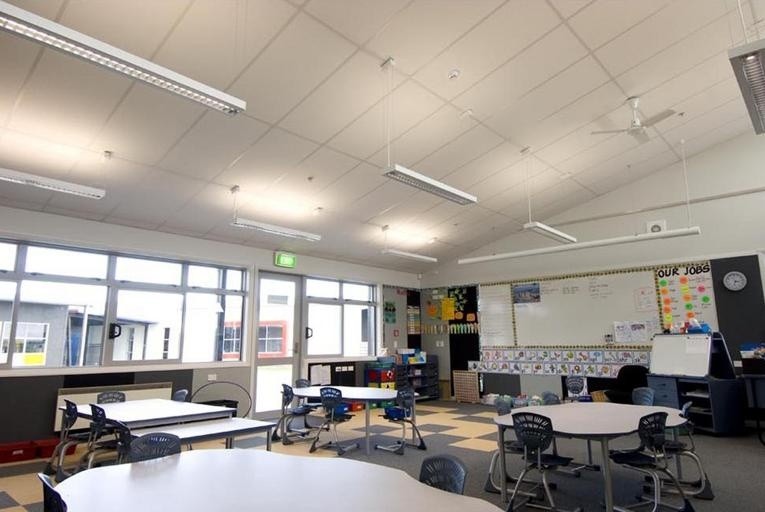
xmin=409 ymin=369 xmax=423 ymax=388
xmin=381 ymin=347 xmax=403 ymax=365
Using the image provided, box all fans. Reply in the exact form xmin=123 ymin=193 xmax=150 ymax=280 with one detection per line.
xmin=591 ymin=96 xmax=676 ymax=146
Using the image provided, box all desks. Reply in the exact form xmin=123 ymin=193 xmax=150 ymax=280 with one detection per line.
xmin=58 ymin=398 xmax=238 ymax=450
xmin=54 ymin=448 xmax=483 ymax=512
xmin=308 ymin=361 xmax=366 ymax=387
xmin=290 ymin=387 xmax=420 ymax=455
xmin=129 ymin=416 xmax=279 ymax=459
xmin=737 ymin=375 xmax=765 ymax=445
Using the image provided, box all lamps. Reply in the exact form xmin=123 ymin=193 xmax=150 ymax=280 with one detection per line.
xmin=230 ymin=184 xmax=323 ymax=243
xmin=382 ymin=223 xmax=439 ymax=266
xmin=0 ymin=147 xmax=113 ymax=200
xmin=458 ymin=139 xmax=700 ymax=266
xmin=520 ymin=146 xmax=577 ymax=244
xmin=380 ymin=57 xmax=478 ymax=206
xmin=731 ymin=36 xmax=765 ymax=135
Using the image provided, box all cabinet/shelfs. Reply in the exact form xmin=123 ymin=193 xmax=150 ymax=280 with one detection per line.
xmin=364 ymin=354 xmax=440 ymax=402
xmin=646 ymin=374 xmax=747 ymax=435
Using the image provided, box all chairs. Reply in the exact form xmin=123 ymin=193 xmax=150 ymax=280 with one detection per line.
xmin=273 ymin=384 xmax=316 ymax=445
xmin=95 ymin=391 xmax=126 ymax=402
xmin=421 ymin=452 xmax=471 ymax=494
xmin=39 ymin=472 xmax=67 ymax=511
xmin=485 ymin=376 xmax=713 ymax=512
xmin=46 ymin=400 xmax=96 ymax=473
xmin=74 ymin=403 xmax=123 ymax=472
xmin=123 ymin=433 xmax=178 ymax=463
xmin=309 ymin=387 xmax=361 ymax=458
xmin=377 ymin=388 xmax=428 ymax=454
xmin=1 ymin=0 xmax=246 ymax=117
xmin=605 ymin=365 xmax=649 ymax=404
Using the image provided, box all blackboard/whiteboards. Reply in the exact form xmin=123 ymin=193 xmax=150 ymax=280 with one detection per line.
xmin=477 ymin=259 xmax=724 ymax=353
xmin=649 ymin=333 xmax=713 ymax=379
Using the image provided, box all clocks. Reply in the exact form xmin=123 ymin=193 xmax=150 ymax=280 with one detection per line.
xmin=723 ymin=271 xmax=747 ymax=292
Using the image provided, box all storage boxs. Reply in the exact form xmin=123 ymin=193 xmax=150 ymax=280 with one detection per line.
xmin=0 ymin=441 xmax=36 ymax=463
